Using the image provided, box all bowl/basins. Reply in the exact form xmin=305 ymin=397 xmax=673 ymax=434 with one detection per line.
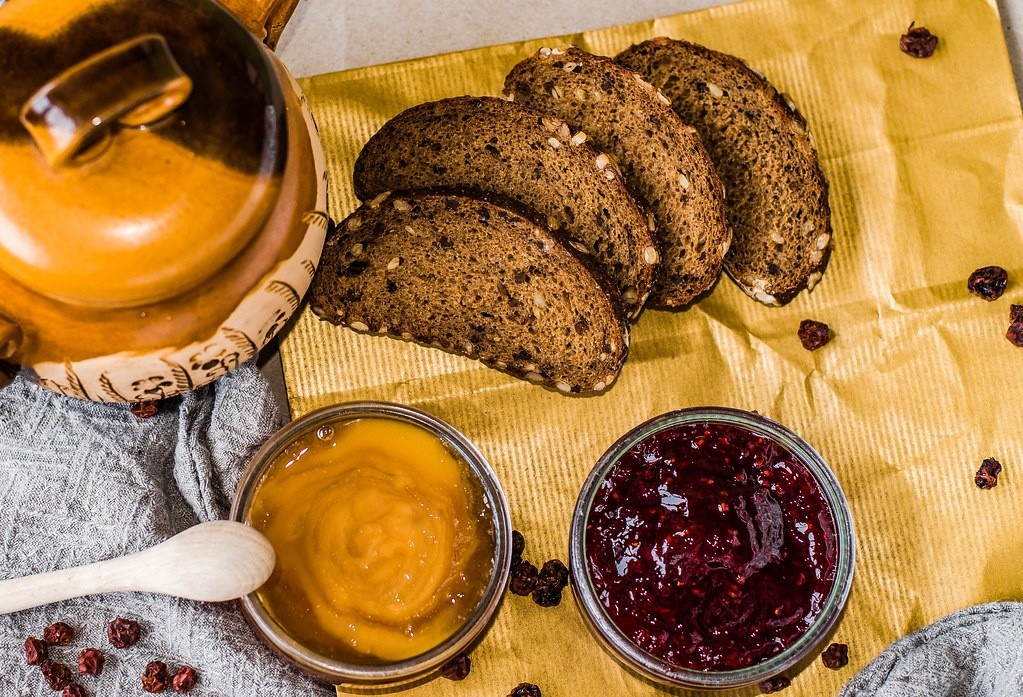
xmin=569 ymin=406 xmax=856 ymax=691
xmin=226 ymin=401 xmax=513 ymax=689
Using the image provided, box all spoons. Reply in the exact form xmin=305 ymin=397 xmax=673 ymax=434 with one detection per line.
xmin=0 ymin=521 xmax=276 ymax=614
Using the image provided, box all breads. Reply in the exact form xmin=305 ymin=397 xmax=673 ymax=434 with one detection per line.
xmin=613 ymin=37 xmax=833 ymax=307
xmin=503 ymin=45 xmax=732 ymax=313
xmin=305 ymin=191 xmax=628 ymax=394
xmin=350 ymin=93 xmax=661 ymax=322
